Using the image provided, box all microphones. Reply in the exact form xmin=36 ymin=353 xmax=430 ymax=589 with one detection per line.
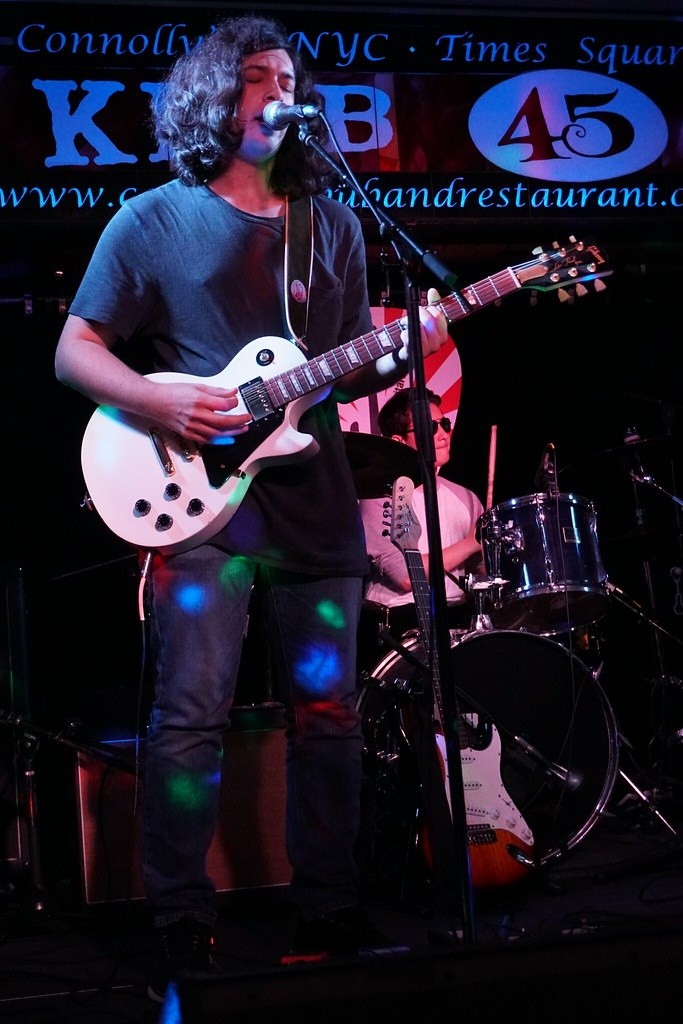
xmin=564 ymin=770 xmax=586 ymax=794
xmin=263 ymin=100 xmax=322 ymax=131
xmin=535 ymin=445 xmax=553 ymax=493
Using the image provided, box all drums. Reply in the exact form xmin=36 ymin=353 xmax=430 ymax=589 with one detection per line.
xmin=472 ymin=490 xmax=614 ymax=638
xmin=353 ymin=625 xmax=621 ymax=874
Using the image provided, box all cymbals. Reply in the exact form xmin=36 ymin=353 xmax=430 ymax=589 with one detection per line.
xmin=341 ymin=426 xmax=426 ymax=501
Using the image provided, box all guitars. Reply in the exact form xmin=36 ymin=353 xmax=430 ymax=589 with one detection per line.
xmin=378 ymin=473 xmax=537 ymax=893
xmin=77 ymin=232 xmax=617 ymax=562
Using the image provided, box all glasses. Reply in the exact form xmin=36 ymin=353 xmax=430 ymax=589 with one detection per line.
xmin=400 ymin=417 xmax=452 ymax=434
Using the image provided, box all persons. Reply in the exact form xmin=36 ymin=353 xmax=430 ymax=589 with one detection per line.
xmin=359 ymin=388 xmax=491 ymax=629
xmin=54 ymin=16 xmax=449 ymax=977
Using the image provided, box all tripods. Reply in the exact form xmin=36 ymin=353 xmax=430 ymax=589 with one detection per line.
xmin=606 ymin=537 xmax=683 ymax=832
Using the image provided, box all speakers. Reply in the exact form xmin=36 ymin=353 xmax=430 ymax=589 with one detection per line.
xmin=70 ymin=703 xmax=294 ymax=904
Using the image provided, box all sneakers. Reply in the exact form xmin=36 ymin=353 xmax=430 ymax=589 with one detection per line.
xmin=145 ymin=924 xmax=211 ymax=1003
xmin=287 ymin=899 xmax=407 ymax=963
